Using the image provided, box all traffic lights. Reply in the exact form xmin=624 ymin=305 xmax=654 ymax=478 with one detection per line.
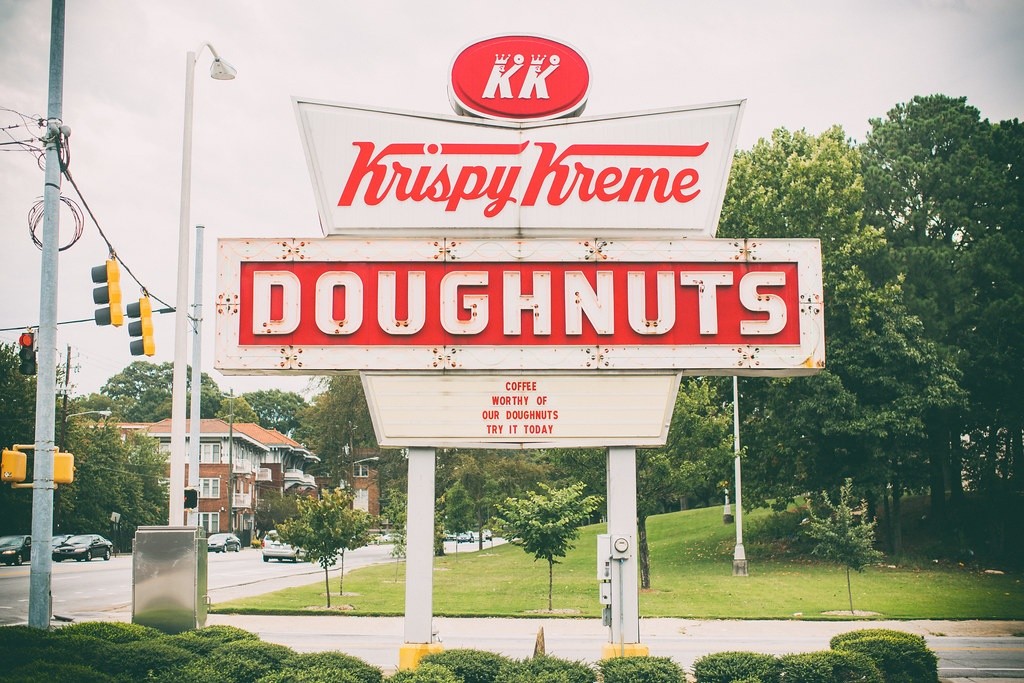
xmin=184 ymin=488 xmax=198 ymax=511
xmin=18 ymin=332 xmax=36 ymax=375
xmin=127 ymin=297 xmax=155 ymax=357
xmin=0 ymin=447 xmax=27 ymax=482
xmin=91 ymin=260 xmax=124 ymax=329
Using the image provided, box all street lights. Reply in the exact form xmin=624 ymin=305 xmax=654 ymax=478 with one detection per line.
xmin=169 ymin=42 xmax=237 ymax=526
xmin=53 ymin=410 xmax=111 ymax=534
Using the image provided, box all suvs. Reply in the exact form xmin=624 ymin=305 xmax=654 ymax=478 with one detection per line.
xmin=262 ymin=529 xmax=307 ymax=563
xmin=472 ymin=531 xmax=486 ymax=541
xmin=443 ymin=530 xmax=456 ymax=541
xmin=483 ymin=529 xmax=493 ymax=540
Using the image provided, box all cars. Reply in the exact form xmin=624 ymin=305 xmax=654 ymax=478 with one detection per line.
xmin=379 ymin=533 xmax=406 ymax=541
xmin=457 ymin=532 xmax=474 ymax=543
xmin=51 ymin=535 xmax=74 ymax=554
xmin=207 ymin=533 xmax=241 ymax=553
xmin=54 ymin=534 xmax=114 ymax=563
xmin=0 ymin=535 xmax=31 ymax=566
